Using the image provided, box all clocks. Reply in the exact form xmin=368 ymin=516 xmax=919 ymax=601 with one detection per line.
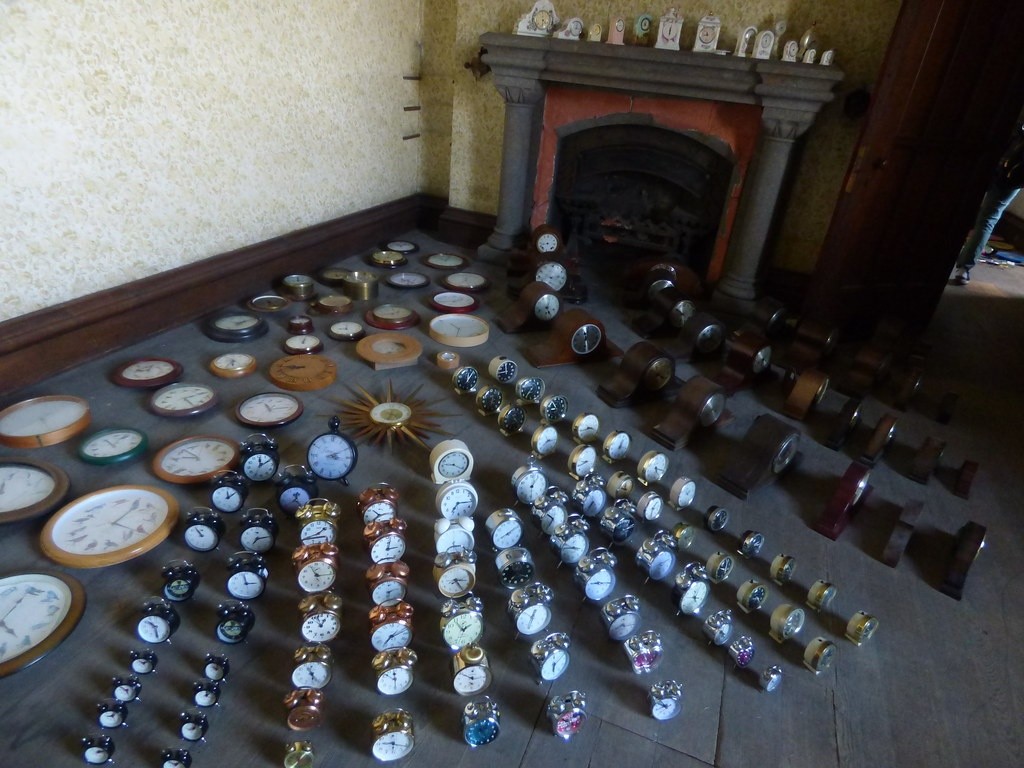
xmin=633 ymin=14 xmax=653 ymax=46
xmin=655 ymin=8 xmax=685 ymax=51
xmin=691 ymin=11 xmax=722 ymax=53
xmin=0 ymin=568 xmax=87 ymax=679
xmin=0 ymin=457 xmax=72 ymax=525
xmin=0 ymin=394 xmax=90 ymax=449
xmin=39 ymin=485 xmax=179 ymax=568
xmin=715 ymin=0 xmax=837 ymax=67
xmin=80 ymin=224 xmax=989 ymax=768
xmin=606 ymin=17 xmax=625 ymax=45
xmin=587 ymin=23 xmax=602 ymax=41
xmin=552 ymin=18 xmax=584 ymax=41
xmin=511 ymin=0 xmax=560 ymax=38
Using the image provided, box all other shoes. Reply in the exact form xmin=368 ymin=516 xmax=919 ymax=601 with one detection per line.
xmin=955 ymin=265 xmax=971 ymax=285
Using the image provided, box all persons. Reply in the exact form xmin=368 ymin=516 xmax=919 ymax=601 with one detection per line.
xmin=954 ymin=105 xmax=1024 ymax=285
